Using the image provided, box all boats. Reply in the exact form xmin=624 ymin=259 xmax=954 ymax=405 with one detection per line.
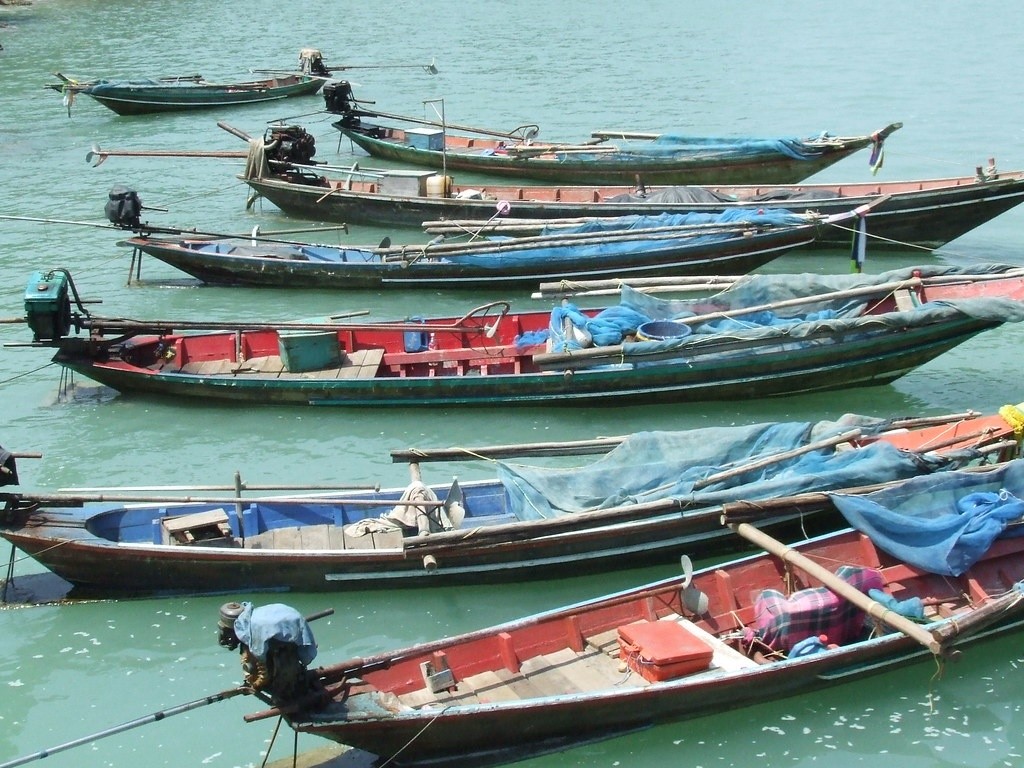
xmin=0 ymin=263 xmax=1024 ymax=457
xmin=86 ymin=117 xmax=1023 ymax=253
xmin=236 ymin=487 xmax=1024 ymax=768
xmin=320 ymin=78 xmax=904 ymax=186
xmin=1 ymin=402 xmax=1024 ymax=616
xmin=42 ymin=47 xmax=335 ymax=116
xmin=0 ymin=175 xmax=894 ymax=294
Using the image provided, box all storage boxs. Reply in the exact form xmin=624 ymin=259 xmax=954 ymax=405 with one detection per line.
xmin=161 ymin=507 xmax=234 ymax=548
xmin=403 ymin=127 xmax=444 ymax=151
xmin=616 ymin=620 xmax=712 ymax=683
xmin=379 ymin=170 xmax=437 ymax=197
xmin=274 ymin=316 xmax=342 ymax=374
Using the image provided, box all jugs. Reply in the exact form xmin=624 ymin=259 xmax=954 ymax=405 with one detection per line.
xmin=402 ymin=316 xmax=428 ymax=352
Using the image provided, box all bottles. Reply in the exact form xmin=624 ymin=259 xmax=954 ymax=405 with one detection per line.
xmin=787 ymin=634 xmax=830 ymax=660
xmin=428 ymin=333 xmax=439 ymax=366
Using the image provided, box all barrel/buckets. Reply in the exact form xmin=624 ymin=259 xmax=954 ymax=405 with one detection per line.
xmin=635 ymin=320 xmax=693 ymax=344
